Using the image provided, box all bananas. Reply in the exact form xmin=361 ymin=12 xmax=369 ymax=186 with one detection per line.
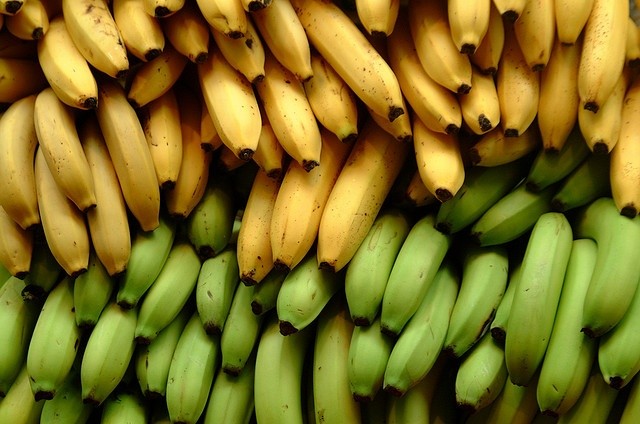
xmin=0 ymin=1 xmax=639 ymax=423
